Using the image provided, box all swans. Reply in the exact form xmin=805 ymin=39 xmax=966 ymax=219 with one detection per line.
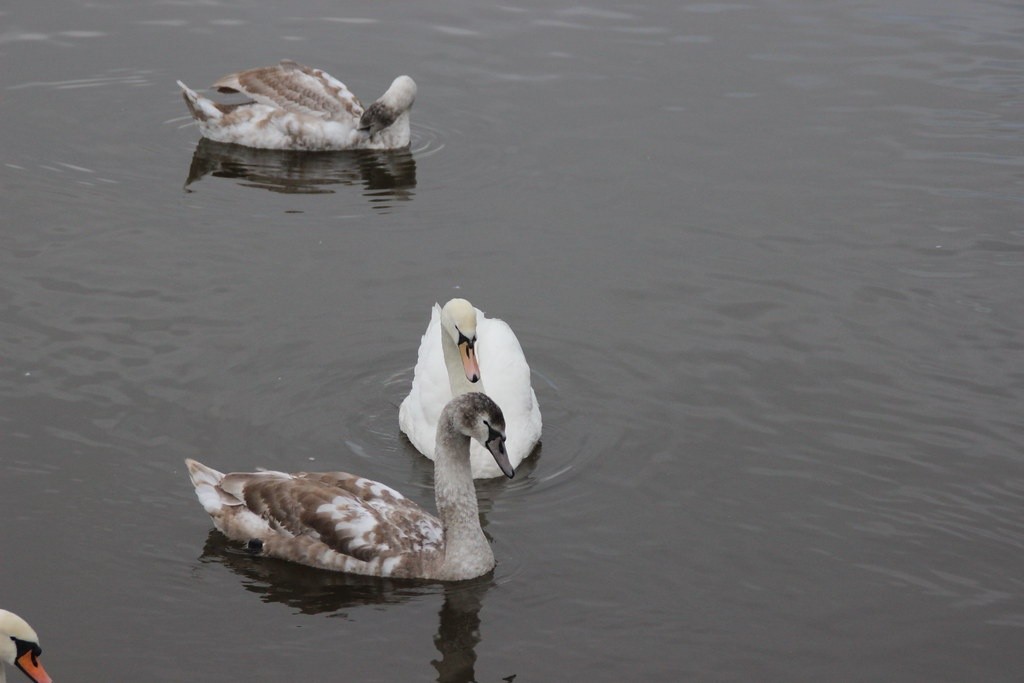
xmin=184 ymin=392 xmax=515 ymax=581
xmin=175 ymin=57 xmax=417 ymax=150
xmin=0 ymin=609 xmax=53 ymax=683
xmin=397 ymin=299 xmax=541 ymax=478
